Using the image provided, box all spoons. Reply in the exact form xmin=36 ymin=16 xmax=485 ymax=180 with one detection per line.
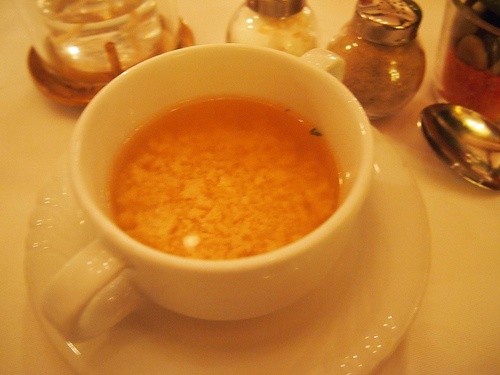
xmin=418 ymin=102 xmax=500 ymax=192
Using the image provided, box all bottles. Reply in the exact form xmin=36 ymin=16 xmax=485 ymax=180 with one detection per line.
xmin=325 ymin=0 xmax=426 ymax=120
xmin=225 ymin=0 xmax=318 ymax=59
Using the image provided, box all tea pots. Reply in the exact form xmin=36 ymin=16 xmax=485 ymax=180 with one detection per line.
xmin=26 ymin=0 xmax=196 ymax=106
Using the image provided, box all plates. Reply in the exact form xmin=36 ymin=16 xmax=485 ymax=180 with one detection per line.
xmin=26 ymin=120 xmax=431 ymax=375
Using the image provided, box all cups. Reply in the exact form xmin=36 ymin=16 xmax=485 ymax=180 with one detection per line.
xmin=41 ymin=45 xmax=375 ymax=340
